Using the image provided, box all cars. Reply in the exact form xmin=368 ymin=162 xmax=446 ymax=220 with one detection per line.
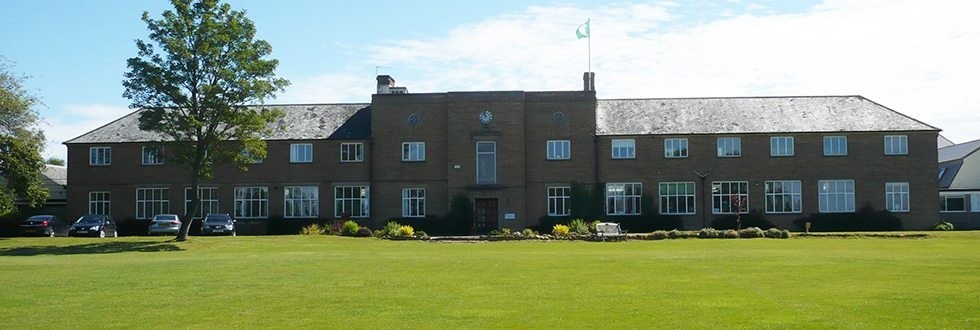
xmin=19 ymin=216 xmax=69 ymax=238
xmin=148 ymin=214 xmax=184 ymax=235
xmin=201 ymin=213 xmax=237 ymax=236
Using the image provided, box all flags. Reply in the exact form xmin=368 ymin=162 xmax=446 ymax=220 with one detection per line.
xmin=576 ymin=19 xmax=591 ymax=39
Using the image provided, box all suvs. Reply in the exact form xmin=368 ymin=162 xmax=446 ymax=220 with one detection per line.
xmin=68 ymin=214 xmax=118 ymax=238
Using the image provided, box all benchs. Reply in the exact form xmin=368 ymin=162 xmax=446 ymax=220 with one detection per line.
xmin=595 ymin=222 xmax=629 ymax=243
